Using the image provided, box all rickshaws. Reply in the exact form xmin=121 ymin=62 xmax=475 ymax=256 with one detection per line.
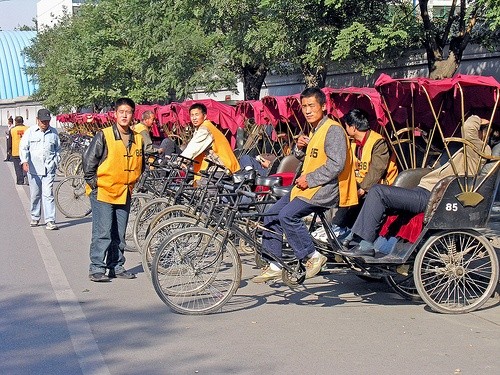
xmin=54 ymin=72 xmax=500 ymax=316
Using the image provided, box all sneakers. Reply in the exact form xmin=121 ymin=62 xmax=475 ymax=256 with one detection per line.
xmin=304 ymin=256 xmax=327 ymax=278
xmin=311 ymin=225 xmax=339 ymax=240
xmin=47 ymin=220 xmax=58 ymax=230
xmin=319 ymin=228 xmax=344 ymax=244
xmin=29 ymin=219 xmax=40 ymax=227
xmin=252 ymin=267 xmax=283 ymax=280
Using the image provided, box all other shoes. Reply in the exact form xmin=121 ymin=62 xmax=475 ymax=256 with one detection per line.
xmin=91 ymin=273 xmax=108 ymax=282
xmin=108 ymin=270 xmax=135 ymax=279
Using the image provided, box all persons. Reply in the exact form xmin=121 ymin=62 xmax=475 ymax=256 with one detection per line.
xmin=252 ymin=87 xmax=359 ymax=283
xmin=4 ymin=116 xmax=14 ymax=161
xmin=341 ymin=110 xmax=492 ymax=257
xmin=19 ymin=109 xmax=60 ymax=230
xmin=80 ymin=98 xmax=146 ymax=281
xmin=129 ymin=110 xmax=163 ymax=213
xmin=236 ymin=139 xmax=291 ymax=179
xmin=8 ymin=115 xmax=29 ymax=184
xmin=174 ymin=103 xmax=241 ymax=206
xmin=312 ymin=108 xmax=400 ymax=241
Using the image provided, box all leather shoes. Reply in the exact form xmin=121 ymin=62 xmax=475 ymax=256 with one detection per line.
xmin=337 ymin=245 xmax=376 ymax=256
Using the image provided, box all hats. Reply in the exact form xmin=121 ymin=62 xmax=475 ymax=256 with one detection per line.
xmin=38 ymin=109 xmax=51 ymax=121
